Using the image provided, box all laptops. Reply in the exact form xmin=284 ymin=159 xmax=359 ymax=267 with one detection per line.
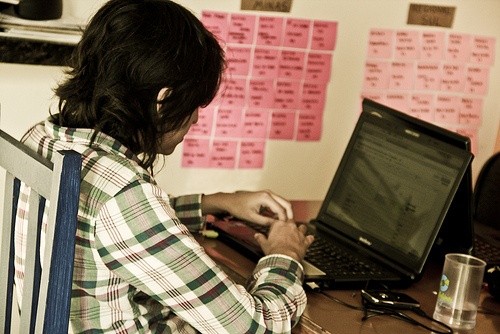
xmin=362 ymin=97 xmax=500 ymax=271
xmin=206 ymin=110 xmax=473 ymax=290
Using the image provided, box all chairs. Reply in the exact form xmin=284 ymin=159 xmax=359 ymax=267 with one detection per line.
xmin=0 ymin=130 xmax=82 ymax=334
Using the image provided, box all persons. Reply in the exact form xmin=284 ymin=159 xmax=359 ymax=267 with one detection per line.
xmin=10 ymin=0 xmax=315 ymax=334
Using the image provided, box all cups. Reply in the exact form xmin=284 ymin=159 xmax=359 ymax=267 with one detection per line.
xmin=432 ymin=253 xmax=486 ymax=330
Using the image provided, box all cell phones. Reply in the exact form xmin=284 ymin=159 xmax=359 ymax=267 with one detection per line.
xmin=360 ymin=289 xmax=421 ymax=308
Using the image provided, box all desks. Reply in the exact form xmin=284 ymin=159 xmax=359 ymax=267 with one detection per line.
xmin=181 ymin=200 xmax=500 ymax=334
